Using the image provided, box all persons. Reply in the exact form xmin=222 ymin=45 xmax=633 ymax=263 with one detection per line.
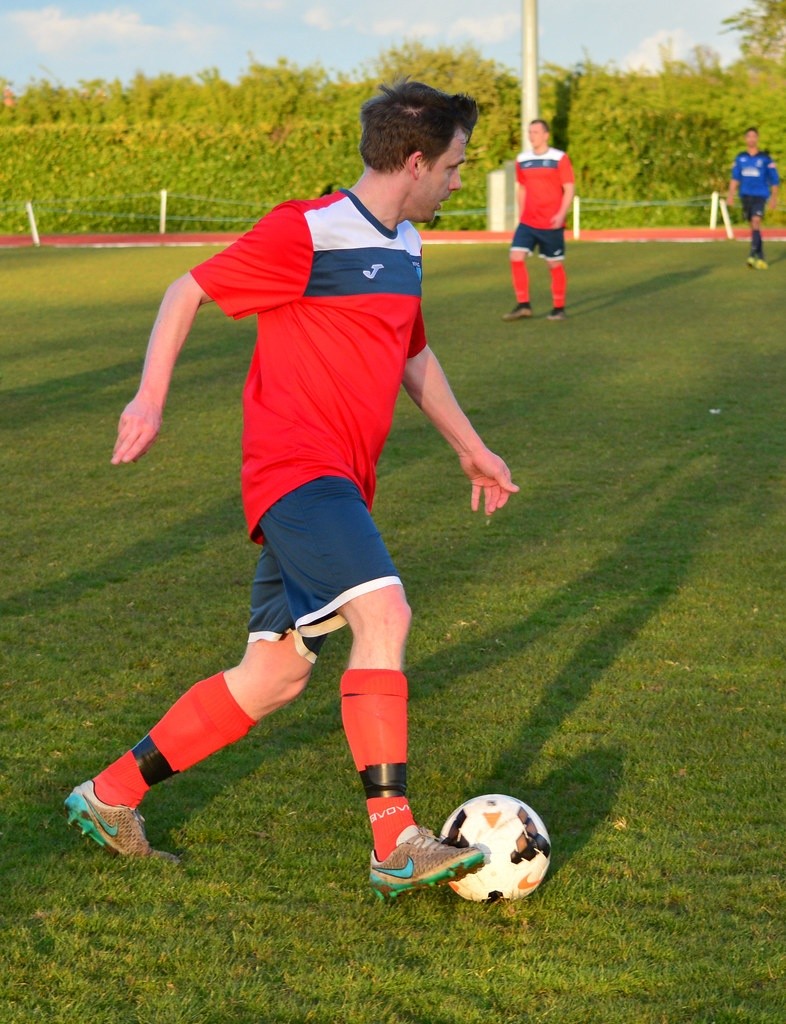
xmin=500 ymin=117 xmax=576 ymax=321
xmin=724 ymin=127 xmax=780 ymax=272
xmin=64 ymin=75 xmax=521 ymax=899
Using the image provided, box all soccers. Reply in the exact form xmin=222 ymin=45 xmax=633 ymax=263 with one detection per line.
xmin=439 ymin=791 xmax=553 ymax=903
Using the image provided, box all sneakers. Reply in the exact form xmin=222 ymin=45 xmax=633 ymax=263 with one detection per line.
xmin=368 ymin=826 xmax=485 ymax=903
xmin=547 ymin=307 xmax=566 ymax=321
xmin=63 ymin=780 xmax=181 ymax=867
xmin=503 ymin=302 xmax=533 ymax=320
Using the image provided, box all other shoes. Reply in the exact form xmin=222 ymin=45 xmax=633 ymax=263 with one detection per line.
xmin=749 ymin=254 xmax=770 ymax=271
